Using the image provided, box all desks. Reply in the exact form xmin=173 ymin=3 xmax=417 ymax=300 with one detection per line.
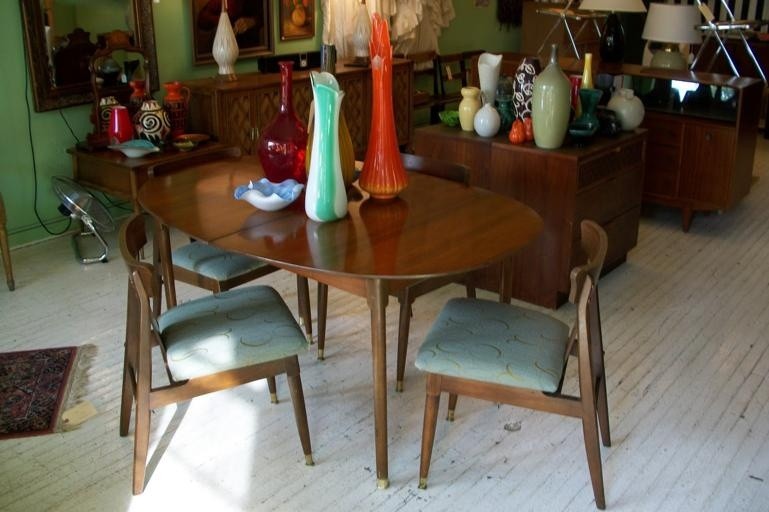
xmin=416 ymin=122 xmax=648 ymax=312
xmin=183 ymin=57 xmax=413 ymax=160
xmin=66 ymin=143 xmax=223 ymax=260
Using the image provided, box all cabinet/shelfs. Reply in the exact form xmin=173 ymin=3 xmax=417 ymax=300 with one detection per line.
xmin=624 ymin=64 xmax=763 ymax=233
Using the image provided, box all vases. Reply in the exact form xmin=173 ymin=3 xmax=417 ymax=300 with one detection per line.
xmin=353 ymin=4 xmax=371 ymax=57
xmin=458 ymin=44 xmax=603 ymax=148
xmin=100 ymin=95 xmax=120 ymax=132
xmin=212 ymin=13 xmax=239 ymax=82
xmin=110 ymin=106 xmax=133 ymax=143
xmin=134 ymin=100 xmax=170 ymax=145
xmin=256 ymin=12 xmax=408 ymax=223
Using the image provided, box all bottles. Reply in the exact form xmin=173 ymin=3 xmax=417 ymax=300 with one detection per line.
xmin=257 ymin=62 xmax=308 ymax=184
xmin=108 ymin=105 xmax=133 ymax=149
xmin=304 ymin=44 xmax=354 ymax=196
xmin=495 ymin=94 xmax=516 ymax=131
xmin=532 ymin=42 xmax=573 ymax=151
xmin=574 ymin=53 xmax=599 ymax=119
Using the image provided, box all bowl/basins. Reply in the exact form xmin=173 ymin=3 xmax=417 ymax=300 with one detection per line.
xmin=438 ymin=110 xmax=460 ymax=128
xmin=233 ymin=179 xmax=306 ymax=213
xmin=168 ymin=134 xmax=211 ymax=153
xmin=108 ymin=140 xmax=158 ymax=159
xmin=237 ymin=208 xmax=304 ymax=244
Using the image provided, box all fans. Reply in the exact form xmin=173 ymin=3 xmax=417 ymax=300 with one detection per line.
xmin=50 ymin=174 xmax=116 ymax=265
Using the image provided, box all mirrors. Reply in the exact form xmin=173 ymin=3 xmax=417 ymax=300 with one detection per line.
xmin=19 ymin=0 xmax=160 ymax=114
xmin=88 ymin=30 xmax=152 ymax=143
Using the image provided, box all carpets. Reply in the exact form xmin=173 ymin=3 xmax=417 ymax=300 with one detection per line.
xmin=0 ymin=344 xmax=97 ymax=440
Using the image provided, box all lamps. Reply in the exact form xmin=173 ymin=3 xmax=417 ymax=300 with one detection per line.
xmin=578 ymin=0 xmax=647 ymax=63
xmin=641 ymin=3 xmax=702 ymax=70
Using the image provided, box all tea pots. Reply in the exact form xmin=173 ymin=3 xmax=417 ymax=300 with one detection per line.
xmin=162 ymin=83 xmax=190 ymax=137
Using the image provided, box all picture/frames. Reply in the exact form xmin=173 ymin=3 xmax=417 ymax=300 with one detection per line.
xmin=279 ymin=0 xmax=315 ymax=41
xmin=190 ymin=0 xmax=275 ymax=67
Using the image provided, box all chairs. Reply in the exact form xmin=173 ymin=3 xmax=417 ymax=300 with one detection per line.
xmin=118 ymin=212 xmax=313 ymax=496
xmin=148 ymin=146 xmax=315 ymax=345
xmin=688 ymin=0 xmax=768 ymax=88
xmin=318 ymin=152 xmax=476 ymax=394
xmin=535 ymin=0 xmax=606 ymax=60
xmin=414 ymin=219 xmax=611 ymax=510
